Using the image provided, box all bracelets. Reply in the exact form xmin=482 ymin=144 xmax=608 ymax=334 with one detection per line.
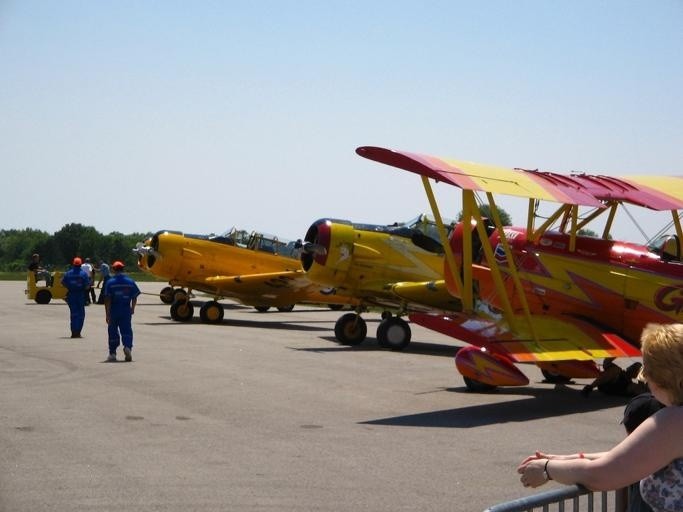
xmin=542 ymin=457 xmax=554 ymax=482
xmin=578 ymin=451 xmax=586 ymax=459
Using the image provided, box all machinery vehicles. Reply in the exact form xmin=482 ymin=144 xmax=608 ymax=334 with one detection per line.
xmin=24 ymin=269 xmax=94 ymax=304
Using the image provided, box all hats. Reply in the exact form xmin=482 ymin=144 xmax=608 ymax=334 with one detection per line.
xmin=624 ymin=394 xmax=666 ymax=431
xmin=73 ymin=257 xmax=81 ymax=264
xmin=112 ymin=262 xmax=124 ymax=269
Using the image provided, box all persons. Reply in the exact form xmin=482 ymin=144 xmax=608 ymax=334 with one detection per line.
xmin=82 ymin=257 xmax=110 ymax=305
xmin=29 ymin=253 xmax=52 ymax=287
xmin=102 ymin=261 xmax=142 ymax=362
xmin=619 ymin=393 xmax=665 ymax=512
xmin=517 ymin=323 xmax=683 ymax=512
xmin=61 ymin=257 xmax=91 ymax=339
xmin=581 ymin=356 xmax=647 ymax=397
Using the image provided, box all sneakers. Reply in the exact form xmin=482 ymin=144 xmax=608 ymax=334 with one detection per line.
xmin=125 ymin=348 xmax=132 ymax=361
xmin=108 ymin=353 xmax=115 ymax=359
